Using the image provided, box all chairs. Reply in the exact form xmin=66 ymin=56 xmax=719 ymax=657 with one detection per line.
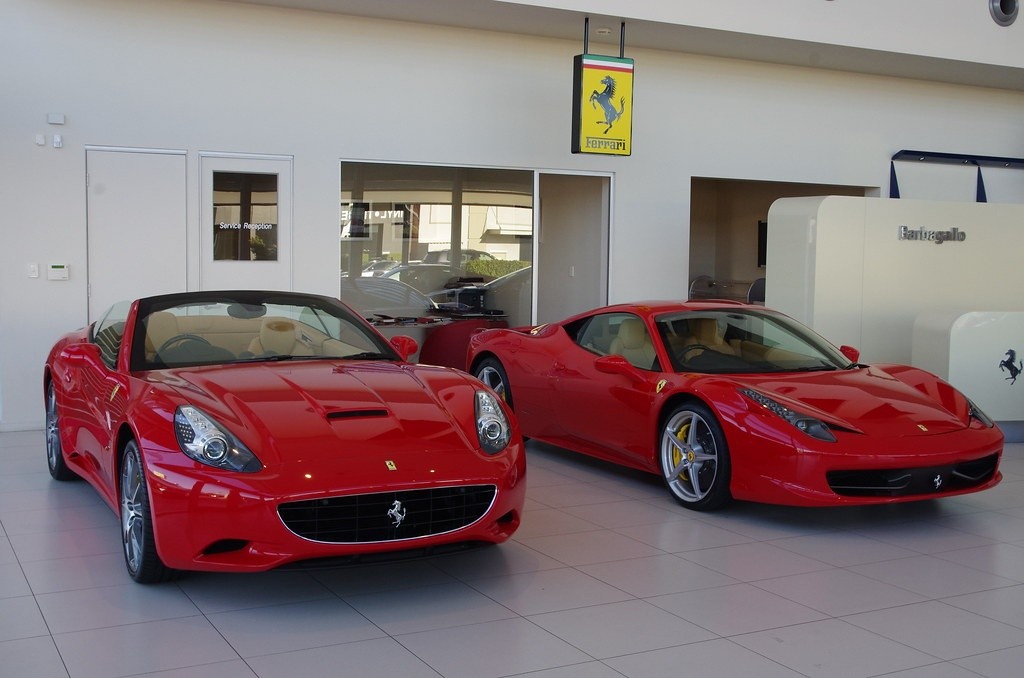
xmin=144 ymin=311 xmax=180 ymax=363
xmin=248 ymin=316 xmax=315 ymax=359
xmin=684 ymin=315 xmax=737 ymax=361
xmin=610 ymin=319 xmax=657 ymax=371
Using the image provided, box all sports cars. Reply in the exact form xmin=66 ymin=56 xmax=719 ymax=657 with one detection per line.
xmin=43 ymin=289 xmax=530 ymax=583
xmin=464 ymin=299 xmax=1006 ymax=515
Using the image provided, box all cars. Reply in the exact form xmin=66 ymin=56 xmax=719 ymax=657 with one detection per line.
xmin=341 ymin=244 xmax=531 ymax=326
